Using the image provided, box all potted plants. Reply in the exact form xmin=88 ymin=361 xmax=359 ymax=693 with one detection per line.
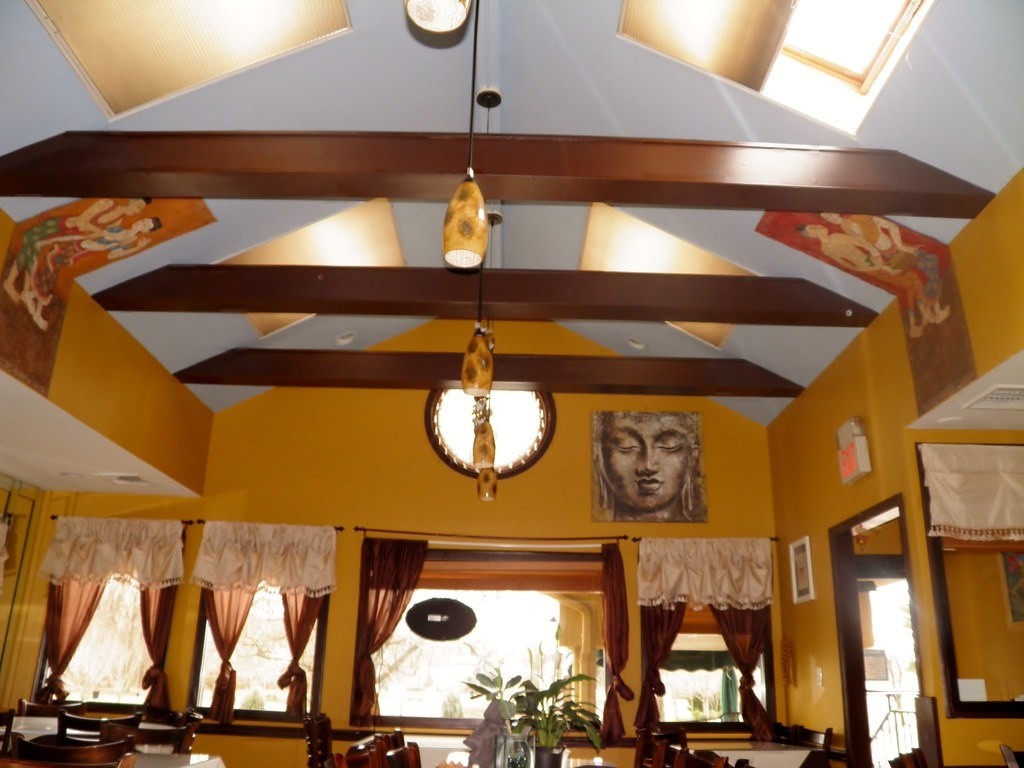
xmin=515 ymin=670 xmax=603 ymax=768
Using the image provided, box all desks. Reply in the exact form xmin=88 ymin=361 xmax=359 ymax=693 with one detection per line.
xmin=667 ymin=740 xmax=833 ymax=768
xmin=415 ymin=747 xmax=619 ymax=768
xmin=0 ymin=716 xmax=176 ymax=743
xmin=130 ymin=750 xmax=227 ymax=768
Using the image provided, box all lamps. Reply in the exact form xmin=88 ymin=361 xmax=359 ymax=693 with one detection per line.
xmin=405 ymin=0 xmax=498 ymax=506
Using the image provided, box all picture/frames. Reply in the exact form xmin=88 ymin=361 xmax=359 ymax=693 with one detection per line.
xmin=789 ymin=536 xmax=815 ymax=604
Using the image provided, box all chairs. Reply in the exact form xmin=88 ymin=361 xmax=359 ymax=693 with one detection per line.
xmin=633 ymin=724 xmax=751 ymax=768
xmin=303 ymin=713 xmax=422 ymax=768
xmin=772 ymin=721 xmax=833 ymax=768
xmin=0 ymin=695 xmax=204 ymax=768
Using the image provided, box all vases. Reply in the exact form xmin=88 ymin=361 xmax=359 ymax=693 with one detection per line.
xmin=495 ymin=731 xmax=537 ymax=768
